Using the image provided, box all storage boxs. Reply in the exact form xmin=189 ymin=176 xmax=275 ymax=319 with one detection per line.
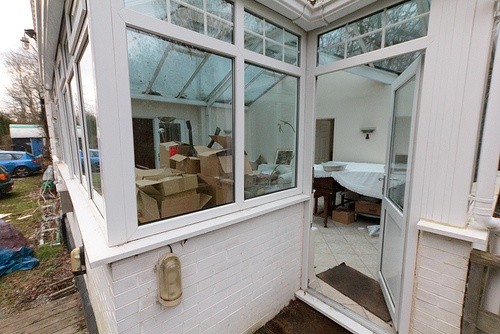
xmin=135 ymin=134 xmax=258 ymax=222
xmin=355 ymin=197 xmax=380 ymax=214
xmin=332 ymin=207 xmax=357 ymax=224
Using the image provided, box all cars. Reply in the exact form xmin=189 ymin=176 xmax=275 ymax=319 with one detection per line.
xmin=78 ymin=148 xmax=101 ymax=173
xmin=0 ymin=151 xmax=42 ymax=178
xmin=0 ymin=164 xmax=15 ymax=192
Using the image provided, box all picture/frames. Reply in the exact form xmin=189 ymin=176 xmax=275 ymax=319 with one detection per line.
xmin=274 ymin=150 xmax=294 ymax=166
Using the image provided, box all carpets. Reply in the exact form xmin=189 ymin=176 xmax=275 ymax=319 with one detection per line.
xmin=316 ymin=263 xmax=392 ymax=323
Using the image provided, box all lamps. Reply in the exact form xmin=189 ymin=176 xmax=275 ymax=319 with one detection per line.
xmin=21 ymin=30 xmax=35 ymax=51
xmin=71 ymin=246 xmax=87 ymax=276
xmin=154 ymin=251 xmax=183 ymax=308
xmin=361 ymin=128 xmax=376 ymax=138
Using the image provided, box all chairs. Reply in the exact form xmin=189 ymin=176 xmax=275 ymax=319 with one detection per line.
xmin=314 ymin=175 xmax=350 ymax=227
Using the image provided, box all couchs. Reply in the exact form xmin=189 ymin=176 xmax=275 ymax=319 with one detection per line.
xmin=258 ymin=163 xmax=293 ymax=191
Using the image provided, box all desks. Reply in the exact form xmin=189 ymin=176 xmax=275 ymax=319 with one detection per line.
xmin=313 ymin=162 xmax=387 ymax=220
